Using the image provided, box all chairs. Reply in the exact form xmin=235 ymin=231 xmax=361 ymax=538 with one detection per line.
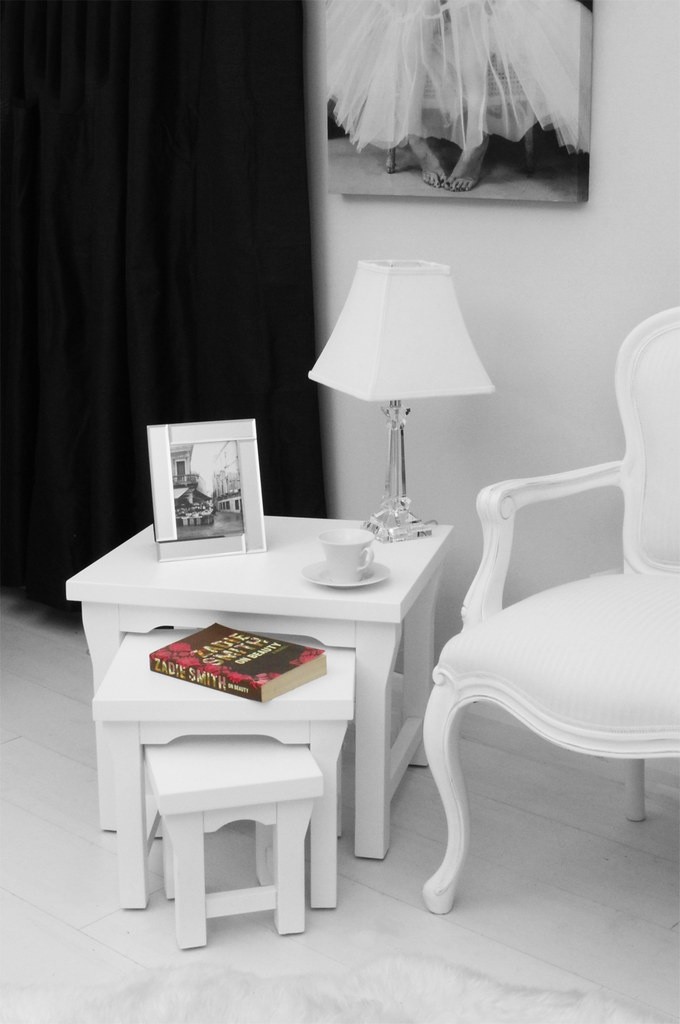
xmin=418 ymin=305 xmax=680 ymax=917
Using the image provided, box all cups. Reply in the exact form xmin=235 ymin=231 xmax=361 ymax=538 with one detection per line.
xmin=319 ymin=530 xmax=374 ymax=582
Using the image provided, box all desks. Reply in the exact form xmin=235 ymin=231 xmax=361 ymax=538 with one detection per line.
xmin=90 ymin=626 xmax=358 ymax=913
xmin=64 ymin=515 xmax=455 ymax=862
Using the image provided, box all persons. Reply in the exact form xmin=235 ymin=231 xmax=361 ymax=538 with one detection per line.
xmin=403 ymin=0 xmax=491 ymax=193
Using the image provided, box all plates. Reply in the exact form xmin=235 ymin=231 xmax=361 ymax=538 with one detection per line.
xmin=301 ymin=560 xmax=390 ymax=587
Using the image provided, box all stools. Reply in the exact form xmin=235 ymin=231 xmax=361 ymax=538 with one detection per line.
xmin=144 ymin=735 xmax=326 ymax=951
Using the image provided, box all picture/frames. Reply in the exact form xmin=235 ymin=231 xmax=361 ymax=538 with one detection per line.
xmin=145 ymin=417 xmax=267 ymax=566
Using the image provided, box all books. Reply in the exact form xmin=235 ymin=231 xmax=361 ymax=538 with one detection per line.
xmin=149 ymin=622 xmax=327 ymax=703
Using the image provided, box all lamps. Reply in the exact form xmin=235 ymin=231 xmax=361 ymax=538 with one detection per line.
xmin=308 ymin=256 xmax=497 ymax=547
